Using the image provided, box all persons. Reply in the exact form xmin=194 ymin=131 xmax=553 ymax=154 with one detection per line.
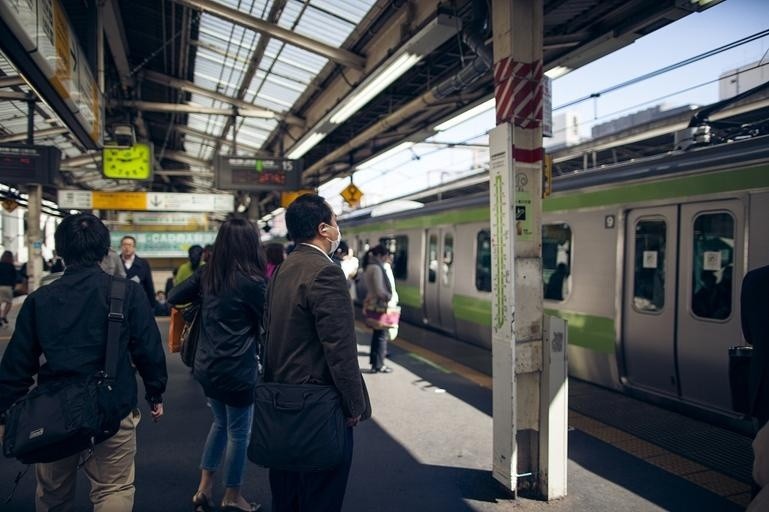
xmin=166 ymin=209 xmax=272 ymax=511
xmin=151 ymin=243 xmax=219 ymax=318
xmin=1 ymin=250 xmax=64 ymax=325
xmin=255 ymin=234 xmax=399 ymax=377
xmin=118 ymin=235 xmax=158 ymax=309
xmin=1 ymin=214 xmax=170 ymax=512
xmin=740 ymin=265 xmax=769 ymax=512
xmin=257 ymin=192 xmax=372 ymax=512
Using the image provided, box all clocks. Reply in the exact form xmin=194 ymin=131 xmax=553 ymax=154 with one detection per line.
xmin=101 ymin=140 xmax=153 ymax=182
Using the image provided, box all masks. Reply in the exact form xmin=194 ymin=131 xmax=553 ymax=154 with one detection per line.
xmin=329 ymin=227 xmax=341 ymax=254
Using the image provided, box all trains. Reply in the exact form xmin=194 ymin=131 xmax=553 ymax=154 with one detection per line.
xmin=325 ymin=136 xmax=769 ymax=435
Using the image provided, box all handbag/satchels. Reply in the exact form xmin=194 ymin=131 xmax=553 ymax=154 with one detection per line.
xmin=168 ymin=299 xmax=203 ymax=367
xmin=364 ymin=304 xmax=402 ymax=331
xmin=247 ymin=381 xmax=345 ymax=473
xmin=5 ymin=366 xmax=120 ymax=464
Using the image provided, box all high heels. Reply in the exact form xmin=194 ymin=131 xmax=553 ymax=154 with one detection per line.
xmin=193 ymin=493 xmax=266 ymax=512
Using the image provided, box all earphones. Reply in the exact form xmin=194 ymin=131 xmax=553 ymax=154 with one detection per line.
xmin=322 ymin=227 xmax=330 ymax=232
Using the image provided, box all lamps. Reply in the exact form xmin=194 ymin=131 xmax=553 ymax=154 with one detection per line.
xmin=284 ymin=15 xmax=464 ymax=161
